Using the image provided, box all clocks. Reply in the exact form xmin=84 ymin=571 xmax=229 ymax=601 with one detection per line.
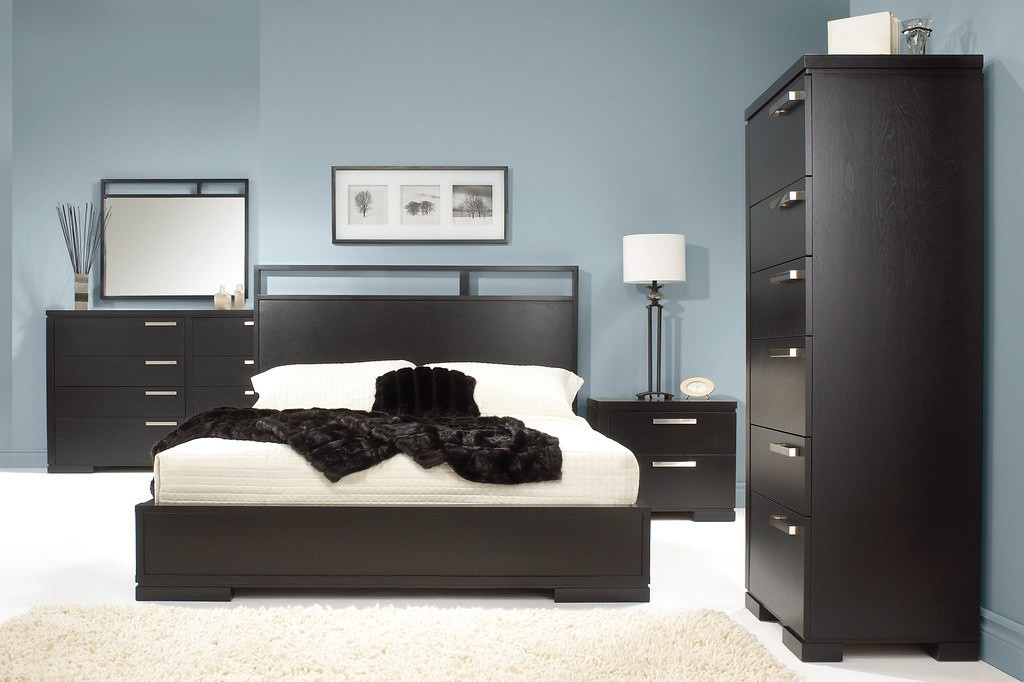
xmin=680 ymin=377 xmax=716 ymax=400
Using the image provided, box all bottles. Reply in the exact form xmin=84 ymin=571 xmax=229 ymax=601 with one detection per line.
xmin=234 ymin=284 xmax=245 ymax=308
xmin=214 ymin=285 xmax=231 ymax=309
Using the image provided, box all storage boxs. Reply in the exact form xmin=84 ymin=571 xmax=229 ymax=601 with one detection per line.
xmin=826 ymin=11 xmax=901 ymax=56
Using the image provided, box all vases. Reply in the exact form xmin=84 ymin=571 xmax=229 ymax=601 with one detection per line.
xmin=73 ymin=272 xmax=89 ymax=312
xmin=902 ymin=18 xmax=933 ymax=55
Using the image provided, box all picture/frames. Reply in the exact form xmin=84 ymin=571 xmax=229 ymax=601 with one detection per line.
xmin=330 ymin=166 xmax=510 ymax=246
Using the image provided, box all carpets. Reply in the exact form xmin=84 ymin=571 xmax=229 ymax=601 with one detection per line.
xmin=0 ymin=604 xmax=805 ymax=682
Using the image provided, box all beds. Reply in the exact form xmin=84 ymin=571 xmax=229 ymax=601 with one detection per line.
xmin=134 ymin=263 xmax=652 ymax=604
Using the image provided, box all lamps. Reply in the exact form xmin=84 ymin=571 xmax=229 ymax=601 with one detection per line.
xmin=622 ymin=233 xmax=687 ymax=401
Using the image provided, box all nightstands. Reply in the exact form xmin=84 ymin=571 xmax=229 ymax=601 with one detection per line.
xmin=587 ymin=397 xmax=737 ymax=523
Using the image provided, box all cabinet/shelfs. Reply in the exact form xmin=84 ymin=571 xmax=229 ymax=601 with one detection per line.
xmin=743 ymin=53 xmax=986 ymax=662
xmin=46 ymin=308 xmax=254 ymax=474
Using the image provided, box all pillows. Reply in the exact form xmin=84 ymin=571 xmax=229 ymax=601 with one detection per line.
xmin=370 ymin=366 xmax=481 ymax=418
xmin=423 ymin=361 xmax=585 ymax=427
xmin=250 ymin=359 xmax=417 ymax=413
